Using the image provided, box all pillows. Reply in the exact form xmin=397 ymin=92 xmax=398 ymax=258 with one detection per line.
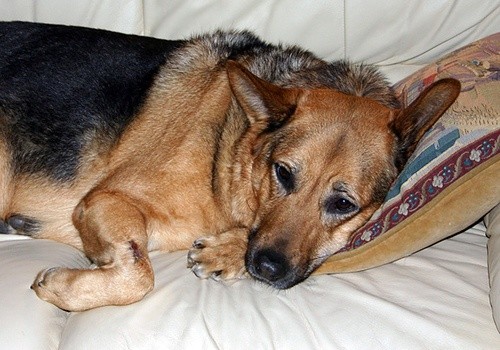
xmin=312 ymin=30 xmax=499 ymax=276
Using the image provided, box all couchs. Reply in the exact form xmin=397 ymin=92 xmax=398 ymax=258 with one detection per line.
xmin=0 ymin=0 xmax=500 ymax=350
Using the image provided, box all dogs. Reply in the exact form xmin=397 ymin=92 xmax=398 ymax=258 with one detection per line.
xmin=0 ymin=18 xmax=463 ymax=312
